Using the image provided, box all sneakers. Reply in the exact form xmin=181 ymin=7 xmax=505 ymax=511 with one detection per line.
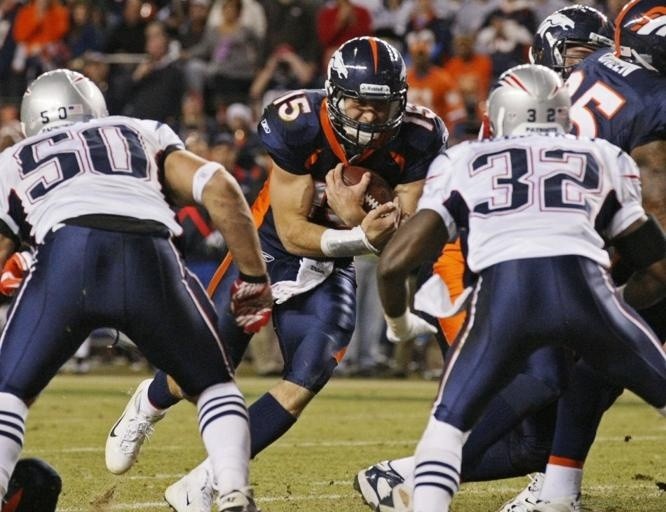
xmin=164 ymin=475 xmax=215 ymax=512
xmin=351 ymin=459 xmax=403 ymax=512
xmin=105 ymin=377 xmax=168 ymax=474
xmin=494 ymin=476 xmax=582 ymax=512
xmin=217 ymin=489 xmax=260 ymax=512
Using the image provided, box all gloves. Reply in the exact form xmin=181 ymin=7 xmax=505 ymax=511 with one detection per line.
xmin=383 ymin=308 xmax=438 ymax=344
xmin=229 ymin=272 xmax=274 ymax=334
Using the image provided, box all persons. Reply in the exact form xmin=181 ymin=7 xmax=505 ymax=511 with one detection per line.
xmin=0 ymin=0 xmax=665 ymax=512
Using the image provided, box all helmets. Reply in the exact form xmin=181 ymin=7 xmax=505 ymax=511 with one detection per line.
xmin=486 ymin=63 xmax=573 ymax=139
xmin=614 ymin=1 xmax=666 ymax=58
xmin=324 ymin=35 xmax=410 ymax=150
xmin=20 ymin=68 xmax=110 ymax=139
xmin=528 ymin=4 xmax=616 ymax=67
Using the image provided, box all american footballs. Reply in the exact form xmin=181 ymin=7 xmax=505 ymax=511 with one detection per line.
xmin=342 ymin=164 xmax=393 ymax=219
